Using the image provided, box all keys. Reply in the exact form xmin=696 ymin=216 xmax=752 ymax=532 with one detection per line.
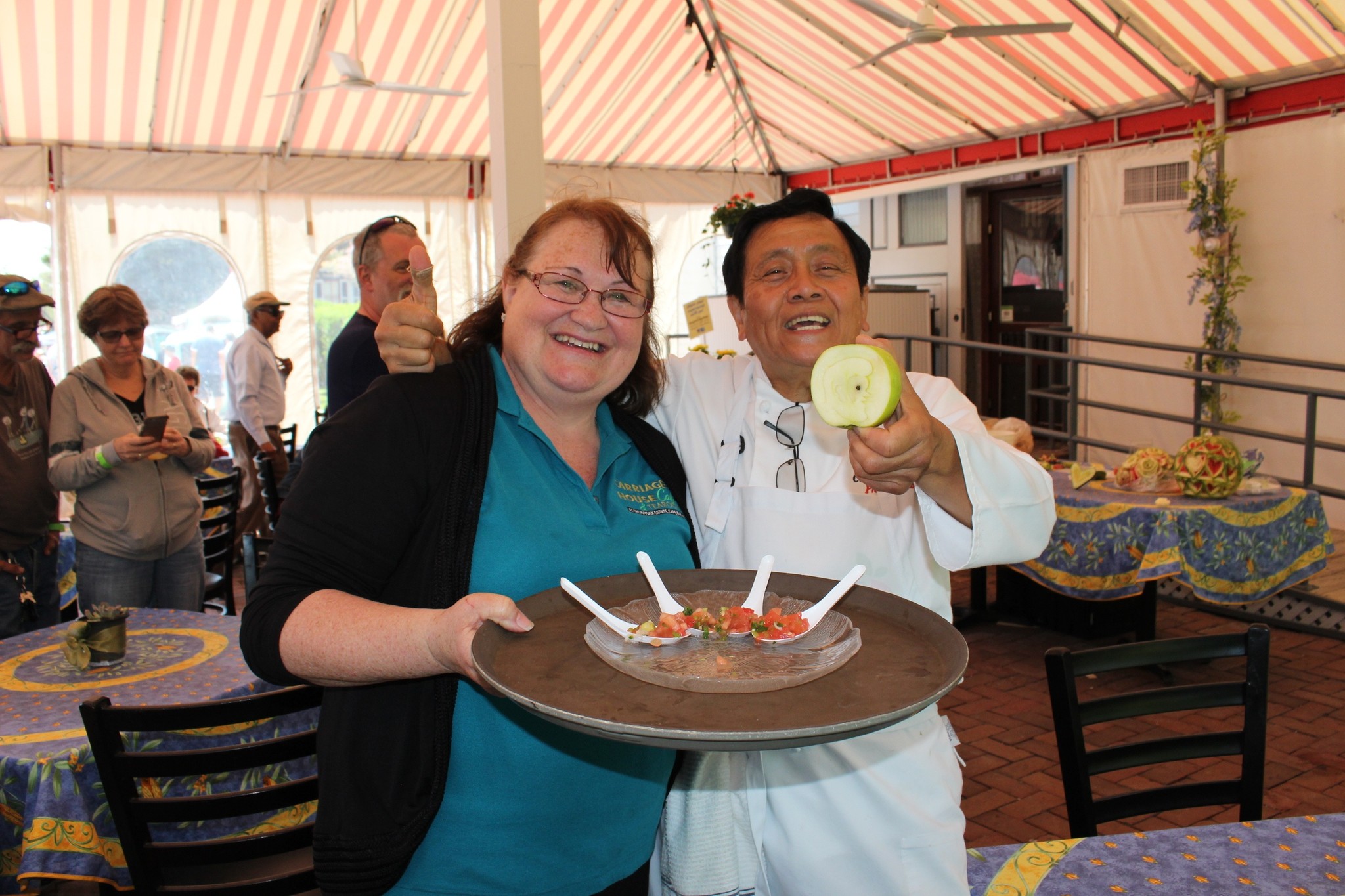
xmin=20 ymin=599 xmax=40 ymax=632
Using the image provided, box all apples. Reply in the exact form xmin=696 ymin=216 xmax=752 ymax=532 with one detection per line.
xmin=811 ymin=344 xmax=901 ymax=430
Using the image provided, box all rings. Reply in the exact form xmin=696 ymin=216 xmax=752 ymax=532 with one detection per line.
xmin=138 ymin=453 xmax=142 ymax=460
xmin=173 ymin=443 xmax=177 ymax=449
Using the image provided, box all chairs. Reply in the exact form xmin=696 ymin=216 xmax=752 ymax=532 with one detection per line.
xmin=242 ymin=531 xmax=274 ymax=604
xmin=252 ymin=452 xmax=279 ymax=531
xmin=196 ymin=467 xmax=241 ymax=616
xmin=78 ymin=683 xmax=321 ymax=896
xmin=1046 ymin=624 xmax=1271 ymax=840
xmin=280 ymin=424 xmax=297 ymax=463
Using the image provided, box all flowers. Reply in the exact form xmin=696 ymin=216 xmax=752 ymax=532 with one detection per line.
xmin=702 ymin=192 xmax=757 ymax=266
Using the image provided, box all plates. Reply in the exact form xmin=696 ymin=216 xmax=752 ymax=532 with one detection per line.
xmin=1239 ymin=449 xmax=1264 ymax=479
xmin=582 ymin=590 xmax=862 ymax=694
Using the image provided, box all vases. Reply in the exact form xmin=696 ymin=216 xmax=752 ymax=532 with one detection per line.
xmin=723 ymin=222 xmax=737 ymax=238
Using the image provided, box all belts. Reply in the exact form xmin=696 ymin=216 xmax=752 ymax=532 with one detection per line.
xmin=266 ymin=424 xmax=280 ymax=431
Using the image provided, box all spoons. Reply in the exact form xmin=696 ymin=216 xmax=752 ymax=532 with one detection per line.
xmin=727 ymin=555 xmax=775 ymax=638
xmin=560 ymin=576 xmax=692 ymax=645
xmin=636 ymin=551 xmax=714 ymax=636
xmin=751 ymin=564 xmax=867 ymax=643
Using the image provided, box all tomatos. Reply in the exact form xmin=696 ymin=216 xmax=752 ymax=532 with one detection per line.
xmin=629 ymin=605 xmax=811 ymax=645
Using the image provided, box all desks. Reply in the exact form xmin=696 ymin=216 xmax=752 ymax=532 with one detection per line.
xmin=59 ymin=457 xmax=233 ymax=609
xmin=959 ymin=459 xmax=1334 ymax=681
xmin=0 ymin=609 xmax=321 ymax=896
xmin=965 ymin=812 xmax=1345 ymax=896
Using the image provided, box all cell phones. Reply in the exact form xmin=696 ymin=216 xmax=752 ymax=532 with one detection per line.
xmin=139 ymin=415 xmax=169 ymax=445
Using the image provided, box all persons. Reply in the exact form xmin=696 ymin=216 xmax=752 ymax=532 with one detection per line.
xmin=326 ymin=215 xmax=430 ymax=420
xmin=47 ymin=284 xmax=216 ymax=616
xmin=225 ymin=291 xmax=293 ymax=568
xmin=175 ymin=365 xmax=219 ymax=432
xmin=0 ymin=273 xmax=63 ymax=641
xmin=373 ymin=187 xmax=1058 ymax=896
xmin=238 ymin=195 xmax=704 ymax=896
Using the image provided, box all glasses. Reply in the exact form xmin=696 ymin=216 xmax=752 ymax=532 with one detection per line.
xmin=0 ymin=281 xmax=41 ymax=296
xmin=187 ymin=385 xmax=197 ymax=391
xmin=254 ymin=307 xmax=280 ymax=317
xmin=359 ymin=216 xmax=417 ymax=265
xmin=0 ymin=317 xmax=52 ymax=341
xmin=93 ymin=324 xmax=144 ymax=343
xmin=762 ymin=403 xmax=806 ymax=494
xmin=519 ymin=269 xmax=653 ymax=318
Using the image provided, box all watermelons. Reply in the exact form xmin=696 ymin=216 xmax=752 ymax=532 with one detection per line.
xmin=1173 ymin=433 xmax=1243 ymax=499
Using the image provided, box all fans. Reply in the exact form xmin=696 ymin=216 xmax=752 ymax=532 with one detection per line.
xmin=848 ymin=0 xmax=1073 ymax=69
xmin=266 ymin=0 xmax=469 ymax=97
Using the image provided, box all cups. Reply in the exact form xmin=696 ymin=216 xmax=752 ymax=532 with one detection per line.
xmin=75 ymin=612 xmax=129 ymax=668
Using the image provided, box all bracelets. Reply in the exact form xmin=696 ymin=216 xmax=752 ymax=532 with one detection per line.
xmin=47 ymin=522 xmax=66 ymax=533
xmin=95 ymin=444 xmax=114 ymax=469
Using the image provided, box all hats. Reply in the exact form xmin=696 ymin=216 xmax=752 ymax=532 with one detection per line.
xmin=0 ymin=275 xmax=54 ymax=309
xmin=244 ymin=292 xmax=291 ymax=312
